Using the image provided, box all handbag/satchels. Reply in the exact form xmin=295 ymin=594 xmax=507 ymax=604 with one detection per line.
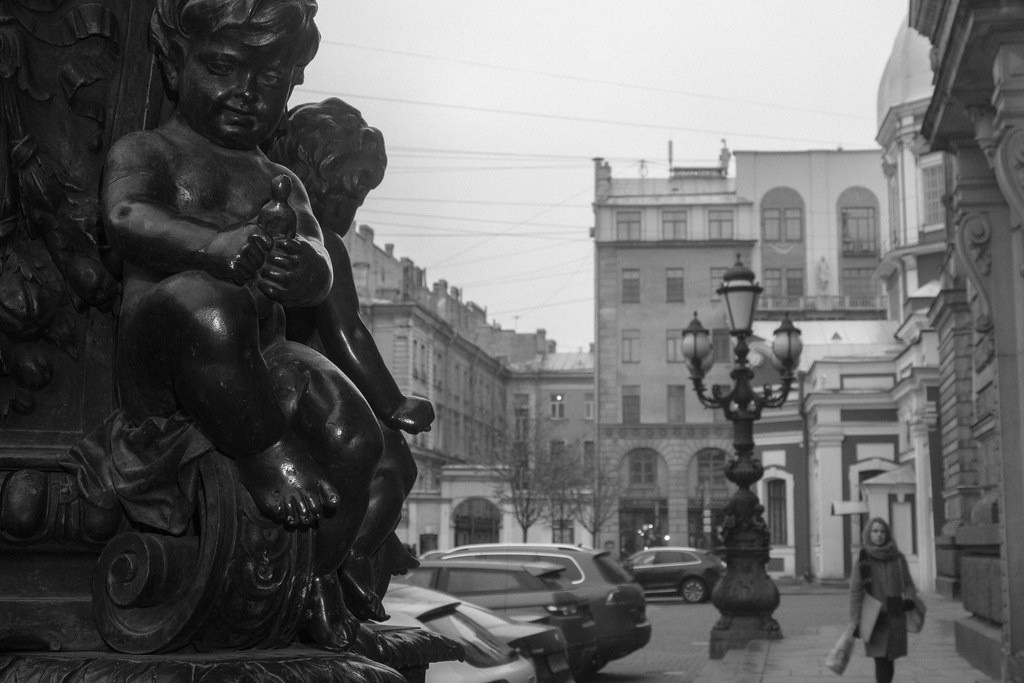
xmin=895 ymin=556 xmax=925 ymax=631
xmin=825 ymin=624 xmax=857 ymax=673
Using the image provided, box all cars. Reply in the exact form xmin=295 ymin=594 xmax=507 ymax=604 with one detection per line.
xmin=377 ymin=585 xmax=537 ymax=683
xmin=395 ymin=559 xmax=599 ymax=673
xmin=387 ymin=583 xmax=575 ymax=682
xmin=616 ymin=546 xmax=729 ymax=598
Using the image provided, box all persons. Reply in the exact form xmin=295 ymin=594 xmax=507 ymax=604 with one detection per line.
xmin=848 ymin=516 xmax=916 ymax=683
xmin=102 ymin=0 xmax=436 ymax=654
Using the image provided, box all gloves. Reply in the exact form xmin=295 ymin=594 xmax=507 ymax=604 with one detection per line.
xmin=904 ymin=600 xmax=914 ymax=611
xmin=853 ymin=627 xmax=859 ymax=637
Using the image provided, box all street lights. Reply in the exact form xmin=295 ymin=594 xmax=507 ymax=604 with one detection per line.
xmin=682 ymin=249 xmax=803 ymax=667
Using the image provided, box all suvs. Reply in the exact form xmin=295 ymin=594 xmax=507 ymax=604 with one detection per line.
xmin=392 ymin=542 xmax=651 ymax=661
